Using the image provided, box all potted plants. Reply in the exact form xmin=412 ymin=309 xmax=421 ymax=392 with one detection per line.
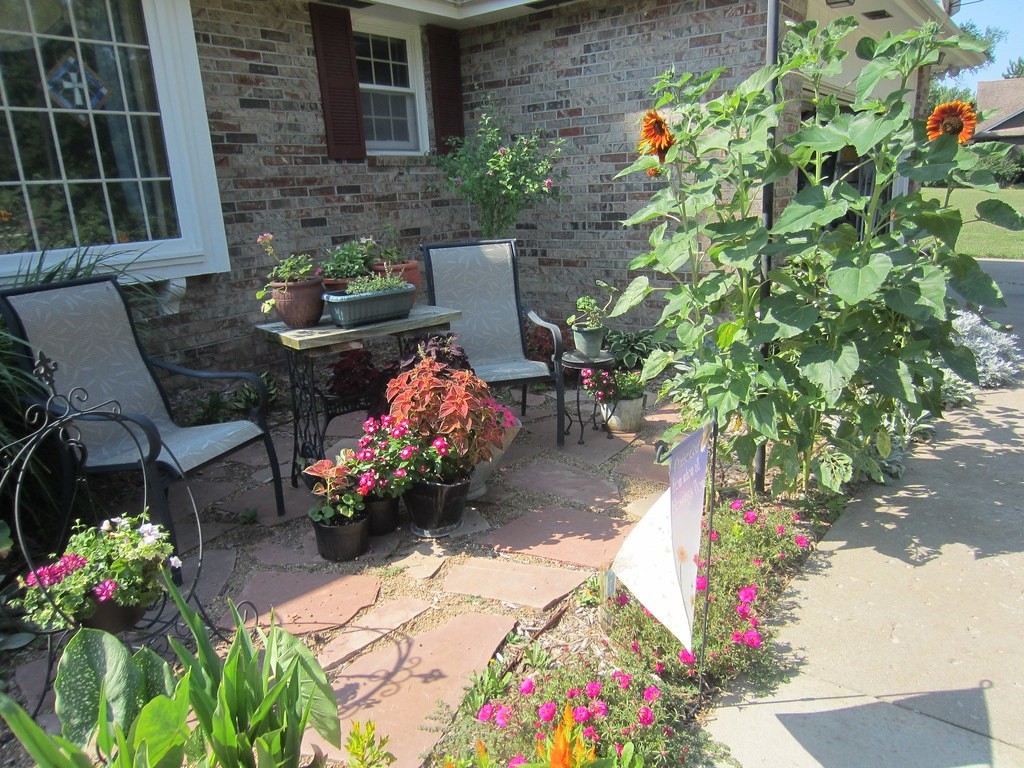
xmin=256 ymin=217 xmax=422 ymax=329
xmin=567 ymin=280 xmax=618 ymax=358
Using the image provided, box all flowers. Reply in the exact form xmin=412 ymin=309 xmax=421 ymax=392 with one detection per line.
xmin=6 ymin=505 xmax=182 ymax=628
xmin=334 ymin=334 xmax=516 ymax=480
xmin=302 ymin=450 xmax=367 ymax=524
xmin=355 ymin=414 xmax=448 ymax=498
xmin=581 ymin=368 xmax=646 ymax=403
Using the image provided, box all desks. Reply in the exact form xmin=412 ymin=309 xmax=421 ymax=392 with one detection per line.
xmin=256 ymin=304 xmax=463 ymax=490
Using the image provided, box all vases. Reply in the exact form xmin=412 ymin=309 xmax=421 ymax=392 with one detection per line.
xmin=401 ymin=477 xmax=471 ymax=533
xmin=317 ymin=522 xmax=368 ymax=562
xmin=73 ymin=597 xmax=146 ymax=635
xmin=363 ymin=494 xmax=400 ymax=534
xmin=599 ymin=398 xmax=642 ymax=433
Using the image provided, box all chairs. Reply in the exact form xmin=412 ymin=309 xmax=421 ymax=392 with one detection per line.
xmin=0 ymin=274 xmax=286 ymax=586
xmin=419 ymin=239 xmax=565 ymax=448
